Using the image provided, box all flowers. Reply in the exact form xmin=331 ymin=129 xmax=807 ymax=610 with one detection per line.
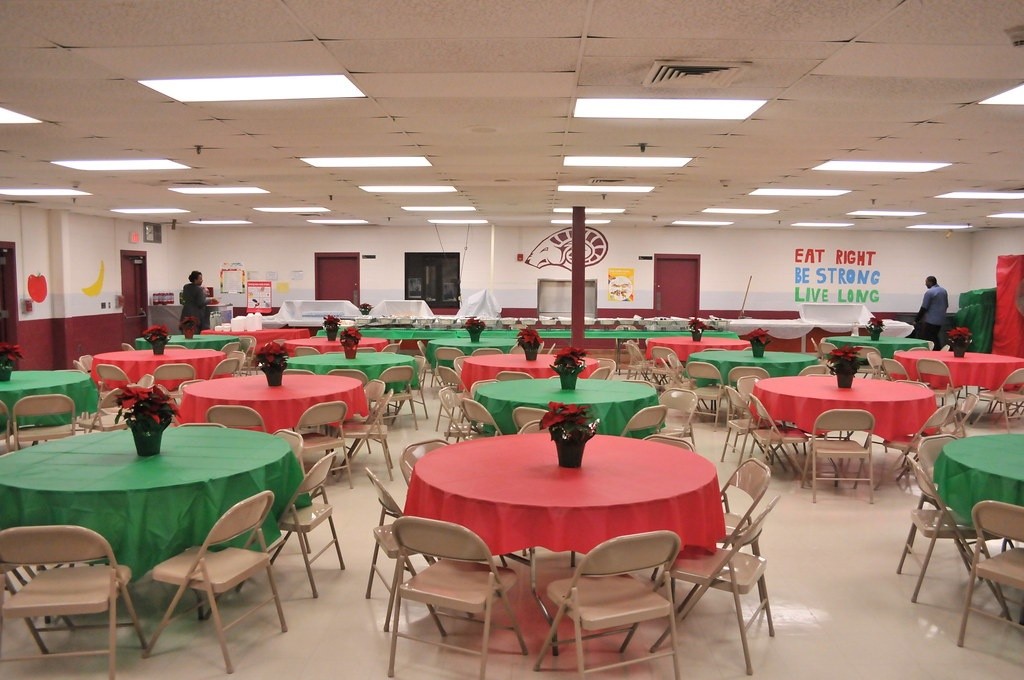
xmin=143 ymin=324 xmax=172 ymax=344
xmin=324 ymin=315 xmax=341 ymax=330
xmin=340 ymin=327 xmax=364 ymax=346
xmin=947 ymin=327 xmax=973 ymax=346
xmin=866 ymin=318 xmax=885 ymax=334
xmin=115 ymin=384 xmax=181 ymax=431
xmin=738 ymin=328 xmax=774 ymax=347
xmin=255 ymin=341 xmax=289 ymax=369
xmin=359 ymin=304 xmax=374 ymax=310
xmin=0 ymin=342 xmax=24 ymax=367
xmin=688 ymin=317 xmax=708 ymax=333
xmin=179 ymin=316 xmax=200 ymax=330
xmin=825 ymin=345 xmax=863 ymax=375
xmin=548 ymin=346 xmax=591 ymax=375
xmin=516 ymin=327 xmax=543 ymax=348
xmin=153 ymin=344 xmax=165 ymax=355
xmin=465 ymin=317 xmax=486 ymax=333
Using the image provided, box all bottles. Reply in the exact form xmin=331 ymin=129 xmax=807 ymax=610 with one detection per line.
xmin=210 ymin=311 xmax=223 ymax=331
xmin=153 ymin=290 xmax=185 ymax=306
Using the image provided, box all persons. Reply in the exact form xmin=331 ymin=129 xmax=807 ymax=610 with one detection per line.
xmin=179 ymin=271 xmax=212 ymax=335
xmin=914 ymin=276 xmax=948 ymax=351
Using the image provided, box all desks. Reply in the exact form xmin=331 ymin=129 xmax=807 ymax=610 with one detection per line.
xmin=91 ymin=350 xmax=234 ymax=430
xmin=173 ymin=374 xmax=370 ymax=475
xmin=426 ymin=337 xmax=519 ymax=370
xmin=0 ymin=370 xmax=99 ymax=447
xmin=683 ymin=350 xmax=819 ymax=422
xmin=472 ymin=379 xmax=666 ymax=440
xmin=200 ymin=329 xmax=311 ymax=354
xmin=457 ymin=354 xmax=600 ymax=392
xmin=134 ymin=334 xmax=243 ymax=352
xmin=933 ymin=433 xmax=1024 ymax=552
xmin=646 ymin=337 xmax=752 ymax=391
xmin=404 ymin=433 xmax=728 ymax=655
xmin=825 ymin=336 xmax=935 ymax=378
xmin=281 ymin=338 xmax=388 ymax=357
xmin=286 ymin=352 xmax=421 ymax=415
xmin=890 ymin=351 xmax=1024 ymax=424
xmin=0 ymin=427 xmax=314 ymax=621
xmin=750 ymin=377 xmax=942 ymax=488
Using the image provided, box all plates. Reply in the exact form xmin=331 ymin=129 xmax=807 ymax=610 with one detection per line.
xmin=231 ymin=315 xmax=246 ymax=332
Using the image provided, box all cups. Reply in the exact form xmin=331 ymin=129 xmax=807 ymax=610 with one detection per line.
xmin=215 ymin=323 xmax=231 ymax=333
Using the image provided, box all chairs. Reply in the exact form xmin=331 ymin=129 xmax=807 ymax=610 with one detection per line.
xmin=0 ymin=328 xmax=1024 ymax=680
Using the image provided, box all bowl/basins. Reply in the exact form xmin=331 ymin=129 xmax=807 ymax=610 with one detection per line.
xmin=246 ymin=312 xmax=263 ymax=332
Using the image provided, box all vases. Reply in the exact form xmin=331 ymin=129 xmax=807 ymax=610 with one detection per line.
xmin=184 ymin=330 xmax=195 ymax=339
xmin=556 ymin=439 xmax=587 ymax=467
xmin=837 ymin=374 xmax=855 ymax=388
xmin=692 ymin=331 xmax=702 ymax=342
xmin=470 ymin=333 xmax=480 ymax=342
xmin=540 ymin=401 xmax=601 ymax=440
xmin=344 ymin=346 xmax=357 ymax=359
xmin=751 ymin=342 xmax=766 ymax=357
xmin=525 ymin=348 xmax=539 ymax=360
xmin=132 ymin=430 xmax=163 ymax=456
xmin=953 ymin=346 xmax=967 ymax=358
xmin=361 ymin=310 xmax=370 ymax=315
xmin=327 ymin=329 xmax=338 ymax=341
xmin=871 ymin=331 xmax=881 ymax=341
xmin=0 ymin=365 xmax=13 ymax=380
xmin=561 ymin=375 xmax=577 ymax=389
xmin=264 ymin=369 xmax=283 ymax=386
